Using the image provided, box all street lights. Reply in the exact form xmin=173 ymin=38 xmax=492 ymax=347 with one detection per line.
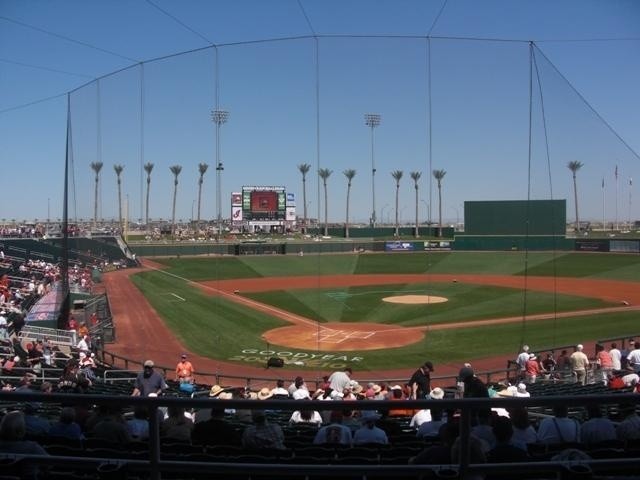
xmin=212 ymin=107 xmax=231 ymax=224
xmin=381 ymin=202 xmax=407 ymax=225
xmin=361 ymin=109 xmax=385 ymax=226
xmin=420 ymin=199 xmax=430 ymax=224
xmin=191 ymin=199 xmax=196 ymax=222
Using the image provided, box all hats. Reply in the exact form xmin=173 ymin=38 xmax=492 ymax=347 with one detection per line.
xmin=181 ymin=354 xmax=187 ymax=358
xmin=258 ymin=387 xmax=275 ymax=401
xmin=371 ymin=385 xmax=383 ymax=394
xmin=528 ymin=353 xmax=537 ymax=360
xmin=143 ymin=359 xmax=154 ymax=369
xmin=455 ymin=366 xmax=474 ymax=382
xmin=429 ymin=387 xmax=445 ymax=399
xmin=208 ymin=384 xmax=225 ymax=396
xmin=352 ymin=384 xmax=364 ymax=394
xmin=424 ymin=362 xmax=434 ymax=372
xmin=390 ymin=384 xmax=402 ymax=391
xmin=292 ymin=389 xmax=311 ymax=400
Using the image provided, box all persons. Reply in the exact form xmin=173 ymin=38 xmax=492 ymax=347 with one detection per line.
xmin=143 ymin=223 xmax=229 ymax=244
xmin=1 ymin=218 xmax=130 ymax=480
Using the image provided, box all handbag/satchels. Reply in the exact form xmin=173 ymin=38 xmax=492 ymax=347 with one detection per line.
xmin=548 ymin=447 xmax=593 ymax=474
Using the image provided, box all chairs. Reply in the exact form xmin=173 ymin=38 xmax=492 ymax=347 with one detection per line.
xmin=2 ymin=345 xmax=640 ymax=480
xmin=0 ymin=232 xmax=138 ymax=346
